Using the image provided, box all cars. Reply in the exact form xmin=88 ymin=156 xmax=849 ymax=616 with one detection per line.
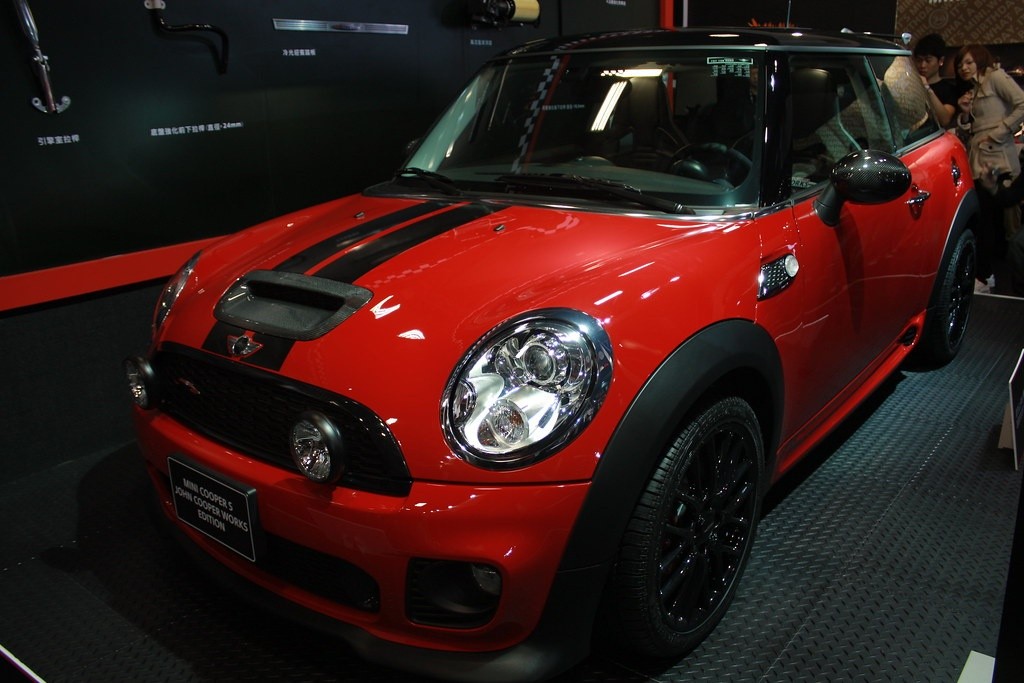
xmin=120 ymin=29 xmax=986 ymax=679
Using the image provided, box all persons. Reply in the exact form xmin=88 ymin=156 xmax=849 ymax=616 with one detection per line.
xmin=954 ymin=44 xmax=1024 ymax=239
xmin=914 ymin=34 xmax=958 ymax=126
xmin=749 ymin=65 xmax=762 ymax=102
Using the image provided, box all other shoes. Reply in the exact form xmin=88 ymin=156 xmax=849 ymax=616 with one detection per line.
xmin=974 ymin=273 xmax=995 ymax=293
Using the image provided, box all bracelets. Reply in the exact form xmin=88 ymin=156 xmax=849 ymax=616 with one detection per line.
xmin=927 ymin=86 xmax=931 ymax=91
